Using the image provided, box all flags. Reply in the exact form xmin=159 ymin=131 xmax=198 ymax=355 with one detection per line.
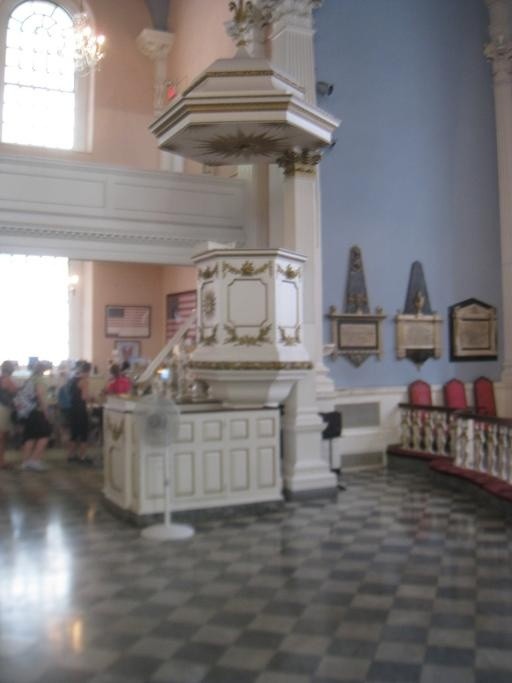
xmin=106 ymin=305 xmax=151 ymax=327
xmin=165 ymin=291 xmax=198 ymax=343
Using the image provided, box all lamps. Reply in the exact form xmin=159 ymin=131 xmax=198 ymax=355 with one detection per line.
xmin=42 ymin=0 xmax=107 ymax=77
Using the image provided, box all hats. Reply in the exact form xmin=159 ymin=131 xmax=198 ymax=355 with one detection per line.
xmin=70 ymin=361 xmax=86 ymax=371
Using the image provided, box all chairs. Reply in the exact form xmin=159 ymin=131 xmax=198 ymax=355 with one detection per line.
xmin=407 ymin=376 xmax=511 ymax=437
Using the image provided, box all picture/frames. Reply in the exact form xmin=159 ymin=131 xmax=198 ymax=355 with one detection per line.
xmin=105 ymin=304 xmax=152 ymax=339
xmin=114 ymin=340 xmax=141 ymax=359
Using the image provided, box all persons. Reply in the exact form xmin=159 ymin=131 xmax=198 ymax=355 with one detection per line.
xmin=1 ymin=354 xmax=132 ymax=474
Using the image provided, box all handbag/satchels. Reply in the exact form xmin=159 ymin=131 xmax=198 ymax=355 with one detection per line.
xmin=11 ymin=379 xmax=38 ymax=419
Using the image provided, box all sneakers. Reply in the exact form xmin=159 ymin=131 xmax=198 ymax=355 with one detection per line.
xmin=68 ymin=456 xmax=93 ymax=465
xmin=20 ymin=459 xmax=48 ymax=472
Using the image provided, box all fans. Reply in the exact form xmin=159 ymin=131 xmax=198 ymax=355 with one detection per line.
xmin=132 ymin=394 xmax=195 ymax=541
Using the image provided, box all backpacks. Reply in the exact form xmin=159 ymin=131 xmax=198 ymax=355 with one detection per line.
xmin=59 ymin=383 xmax=74 ymax=411
